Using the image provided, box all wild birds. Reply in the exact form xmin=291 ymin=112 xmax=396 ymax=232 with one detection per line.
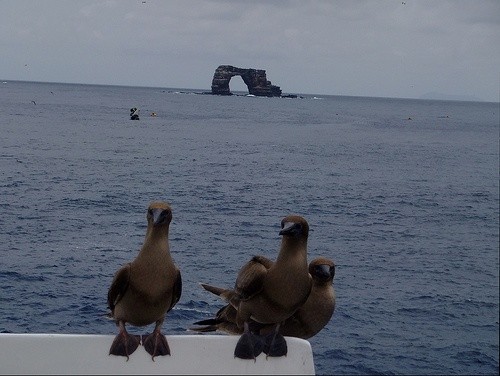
xmin=189 ymin=256 xmax=336 ymax=341
xmin=199 ymin=215 xmax=309 ymax=361
xmin=106 ymin=200 xmax=183 ymax=362
